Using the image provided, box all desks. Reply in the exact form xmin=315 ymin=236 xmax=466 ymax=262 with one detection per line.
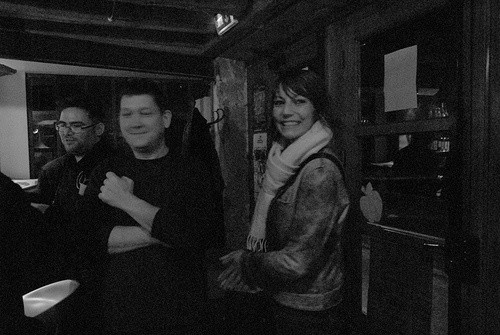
xmin=11 ymin=179 xmax=40 ymax=192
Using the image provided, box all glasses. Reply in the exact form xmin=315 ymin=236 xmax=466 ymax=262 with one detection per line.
xmin=53 ymin=121 xmax=95 ymax=135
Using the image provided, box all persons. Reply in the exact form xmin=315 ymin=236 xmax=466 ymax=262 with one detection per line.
xmin=27 ymin=93 xmax=111 ymax=334
xmin=0 ymin=171 xmax=74 ymax=335
xmin=69 ymin=81 xmax=218 ymax=335
xmin=217 ymin=70 xmax=352 ymax=335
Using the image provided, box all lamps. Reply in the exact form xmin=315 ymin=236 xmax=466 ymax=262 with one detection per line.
xmin=214 ymin=14 xmax=238 ymax=37
